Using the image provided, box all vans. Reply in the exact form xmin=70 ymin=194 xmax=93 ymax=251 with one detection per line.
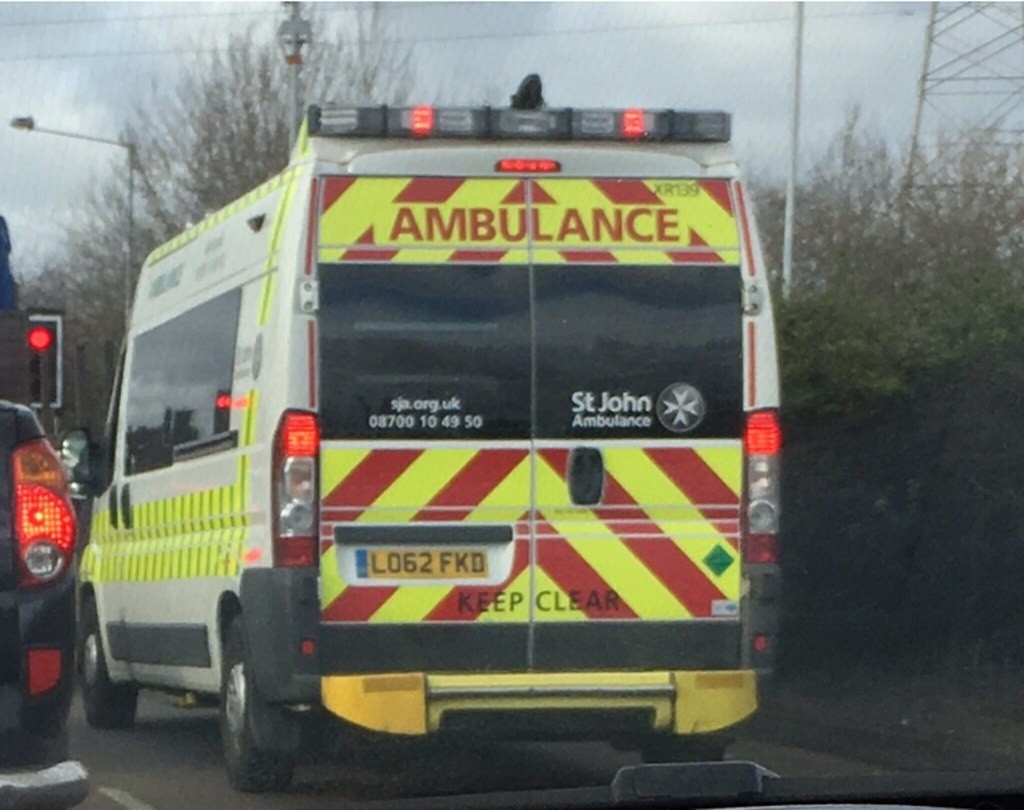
xmin=89 ymin=77 xmax=788 ymax=793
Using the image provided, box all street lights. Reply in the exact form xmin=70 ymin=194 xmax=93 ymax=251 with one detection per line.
xmin=9 ymin=113 xmax=138 ymax=334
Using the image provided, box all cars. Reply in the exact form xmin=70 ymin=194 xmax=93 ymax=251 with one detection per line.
xmin=1 ymin=395 xmax=77 ymax=772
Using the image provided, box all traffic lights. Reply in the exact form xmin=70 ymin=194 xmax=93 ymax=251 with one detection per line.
xmin=28 ymin=314 xmax=62 ymax=409
